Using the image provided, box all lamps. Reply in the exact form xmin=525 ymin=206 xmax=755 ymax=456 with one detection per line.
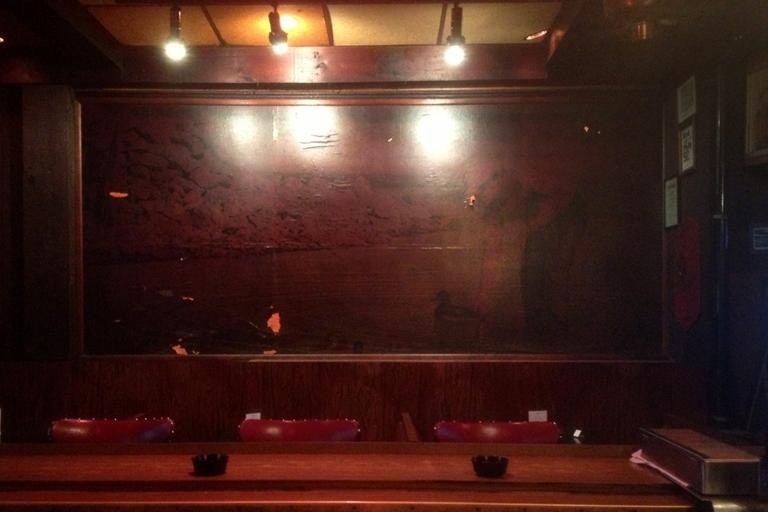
xmin=268 ymin=6 xmax=288 ymax=54
xmin=164 ymin=6 xmax=187 ymax=61
xmin=443 ymin=4 xmax=464 ymax=68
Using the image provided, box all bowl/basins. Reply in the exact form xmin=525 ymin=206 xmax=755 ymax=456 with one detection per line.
xmin=193 ymin=452 xmax=227 ymax=477
xmin=471 ymin=454 xmax=509 ymax=478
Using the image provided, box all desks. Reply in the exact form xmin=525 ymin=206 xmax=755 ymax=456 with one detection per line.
xmin=1 ymin=435 xmax=768 ymax=510
